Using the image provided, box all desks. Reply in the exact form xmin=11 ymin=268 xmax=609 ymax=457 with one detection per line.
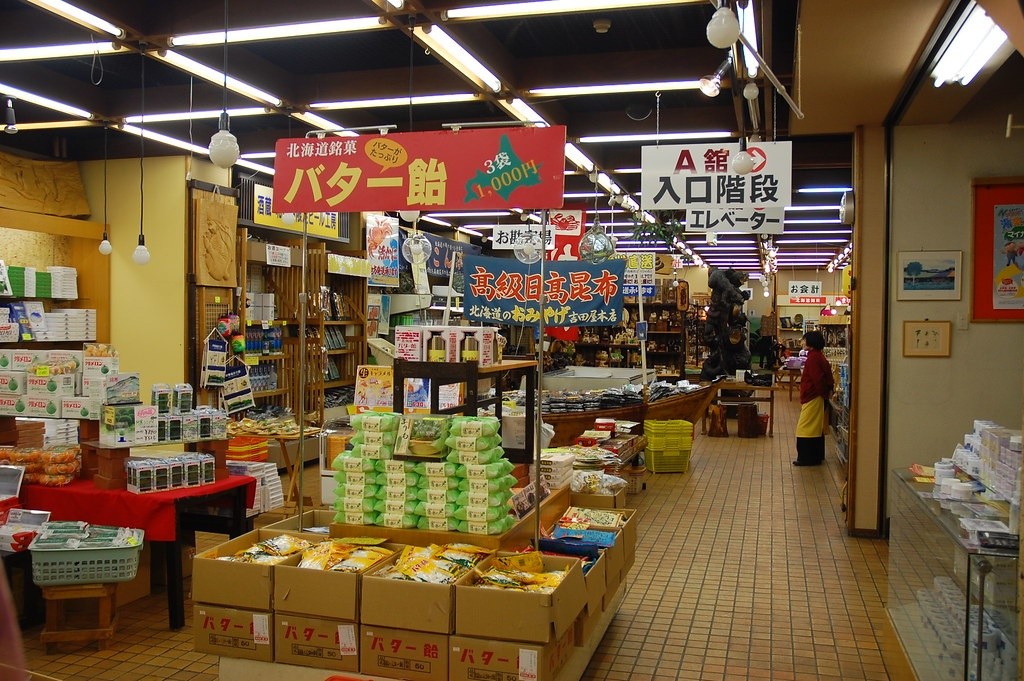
xmin=392 ymin=359 xmax=536 ymax=456
xmin=719 ymin=382 xmax=779 ymax=440
xmin=20 ymin=469 xmax=257 ymax=629
xmin=775 ymin=367 xmax=803 ymax=400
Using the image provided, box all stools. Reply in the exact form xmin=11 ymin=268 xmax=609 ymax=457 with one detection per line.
xmin=41 ymin=583 xmax=119 ymax=655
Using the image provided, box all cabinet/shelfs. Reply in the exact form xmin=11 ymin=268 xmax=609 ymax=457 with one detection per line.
xmin=574 ymin=304 xmax=686 ymax=386
xmin=0 ymin=215 xmax=112 ymax=355
xmin=245 ymin=319 xmax=363 ymax=399
xmin=780 ymin=329 xmax=803 ymax=354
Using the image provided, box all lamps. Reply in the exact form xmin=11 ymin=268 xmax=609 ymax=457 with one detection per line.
xmin=0 ymin=0 xmax=852 ymax=292
xmin=930 ymin=6 xmax=1008 ymax=90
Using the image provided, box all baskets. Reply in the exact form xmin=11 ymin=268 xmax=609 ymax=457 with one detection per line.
xmin=29 ymin=528 xmax=144 ymax=585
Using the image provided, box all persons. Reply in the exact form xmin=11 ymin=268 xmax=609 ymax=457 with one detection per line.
xmin=793 ymin=331 xmax=835 ymax=466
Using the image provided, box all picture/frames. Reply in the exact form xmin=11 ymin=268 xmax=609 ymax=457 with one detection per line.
xmin=903 ymin=322 xmax=951 ymax=358
xmin=968 ymin=178 xmax=1024 ymax=322
xmin=896 ymin=249 xmax=963 ymax=301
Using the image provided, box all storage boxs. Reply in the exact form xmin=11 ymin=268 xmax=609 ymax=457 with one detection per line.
xmin=194 ymin=421 xmax=693 ymax=681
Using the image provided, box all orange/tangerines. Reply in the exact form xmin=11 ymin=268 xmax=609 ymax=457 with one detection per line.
xmin=0 ymin=448 xmax=80 ymax=485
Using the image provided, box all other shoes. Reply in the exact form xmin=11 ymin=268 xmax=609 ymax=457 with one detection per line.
xmin=793 ymin=461 xmax=823 ymax=466
